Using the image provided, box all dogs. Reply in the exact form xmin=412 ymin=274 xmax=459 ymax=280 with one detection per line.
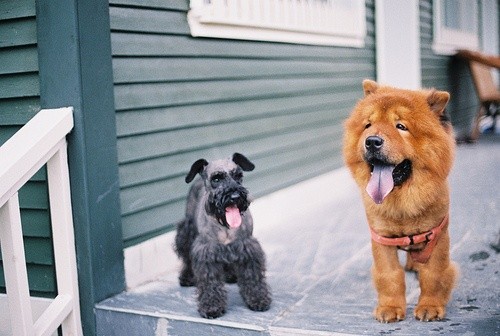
xmin=343 ymin=78 xmax=459 ymax=324
xmin=174 ymin=152 xmax=274 ymax=320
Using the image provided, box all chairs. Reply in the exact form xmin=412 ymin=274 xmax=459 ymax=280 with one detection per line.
xmin=455 ymin=49 xmax=500 ymax=144
